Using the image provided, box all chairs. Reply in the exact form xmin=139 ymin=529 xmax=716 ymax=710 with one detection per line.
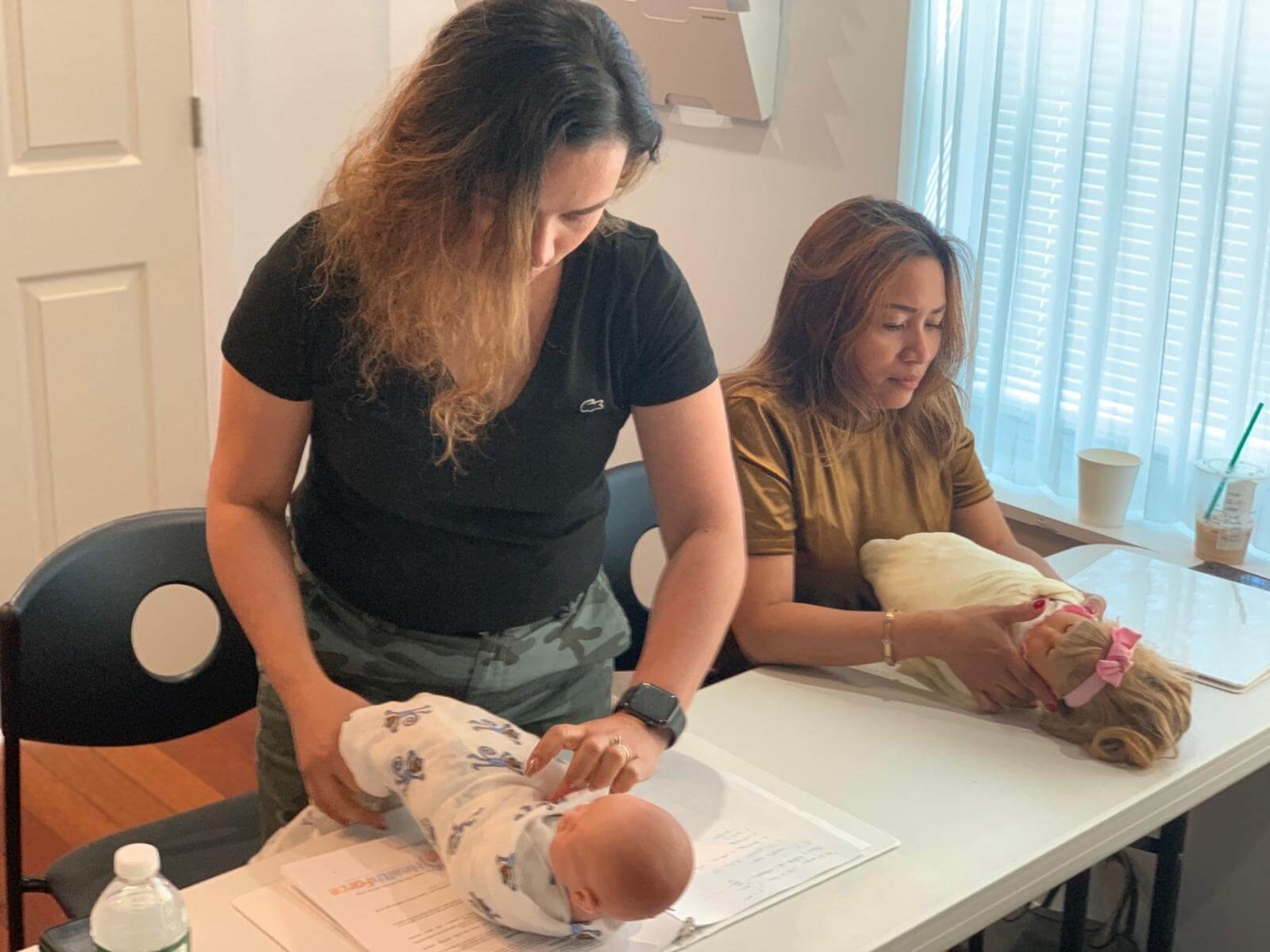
xmin=0 ymin=506 xmax=263 ymax=952
xmin=608 ymin=462 xmax=751 ymax=689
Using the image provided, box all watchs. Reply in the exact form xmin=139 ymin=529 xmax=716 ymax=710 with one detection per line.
xmin=613 ymin=681 xmax=687 ymax=747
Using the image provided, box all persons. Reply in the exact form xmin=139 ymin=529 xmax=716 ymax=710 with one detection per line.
xmin=204 ymin=1 xmax=747 ymax=845
xmin=711 ymin=196 xmax=1108 ymax=713
xmin=339 ymin=692 xmax=696 ymax=942
xmin=860 ymin=532 xmax=1195 ymax=768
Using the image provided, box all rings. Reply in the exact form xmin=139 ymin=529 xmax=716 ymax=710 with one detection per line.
xmin=613 ymin=738 xmax=631 ymax=762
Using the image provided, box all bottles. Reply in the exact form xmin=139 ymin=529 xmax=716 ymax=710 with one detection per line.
xmin=89 ymin=842 xmax=192 ymax=952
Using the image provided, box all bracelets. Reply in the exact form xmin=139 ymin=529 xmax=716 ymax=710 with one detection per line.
xmin=882 ymin=609 xmax=895 ymax=666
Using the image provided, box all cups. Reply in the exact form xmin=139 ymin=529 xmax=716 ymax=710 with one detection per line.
xmin=1193 ymin=456 xmax=1269 ymax=565
xmin=1075 ymin=448 xmax=1144 ymax=529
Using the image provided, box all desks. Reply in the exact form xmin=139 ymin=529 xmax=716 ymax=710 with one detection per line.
xmin=185 ymin=544 xmax=1270 ymax=952
